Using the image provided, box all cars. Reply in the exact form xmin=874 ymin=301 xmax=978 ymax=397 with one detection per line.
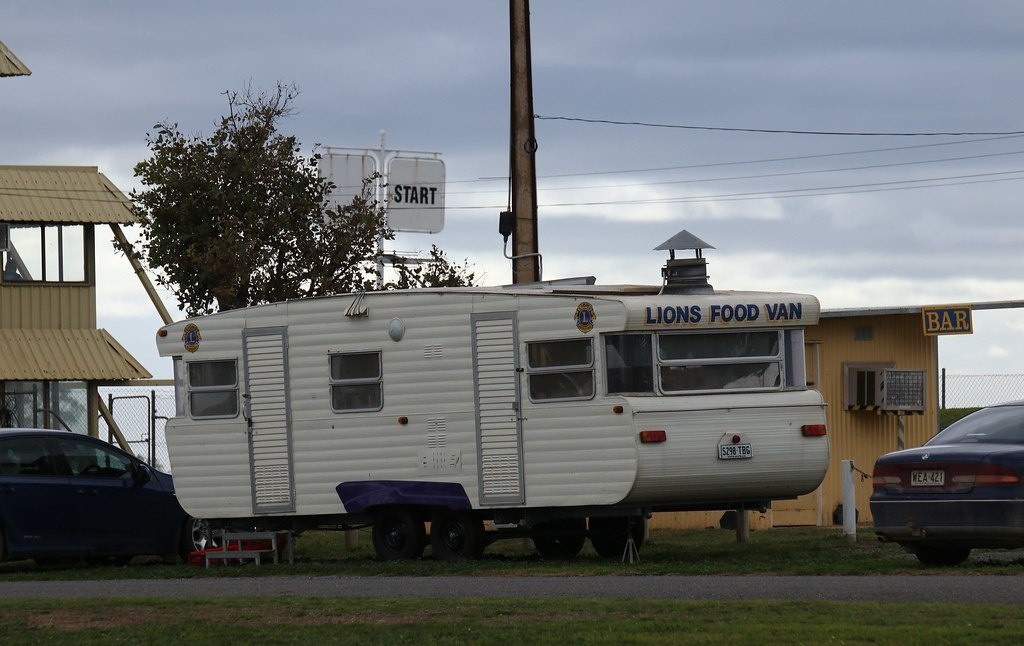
xmin=866 ymin=401 xmax=1024 ymax=568
xmin=0 ymin=425 xmax=233 ymax=568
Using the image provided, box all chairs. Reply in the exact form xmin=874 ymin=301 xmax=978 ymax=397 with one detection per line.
xmin=32 ymin=439 xmax=74 ymax=476
